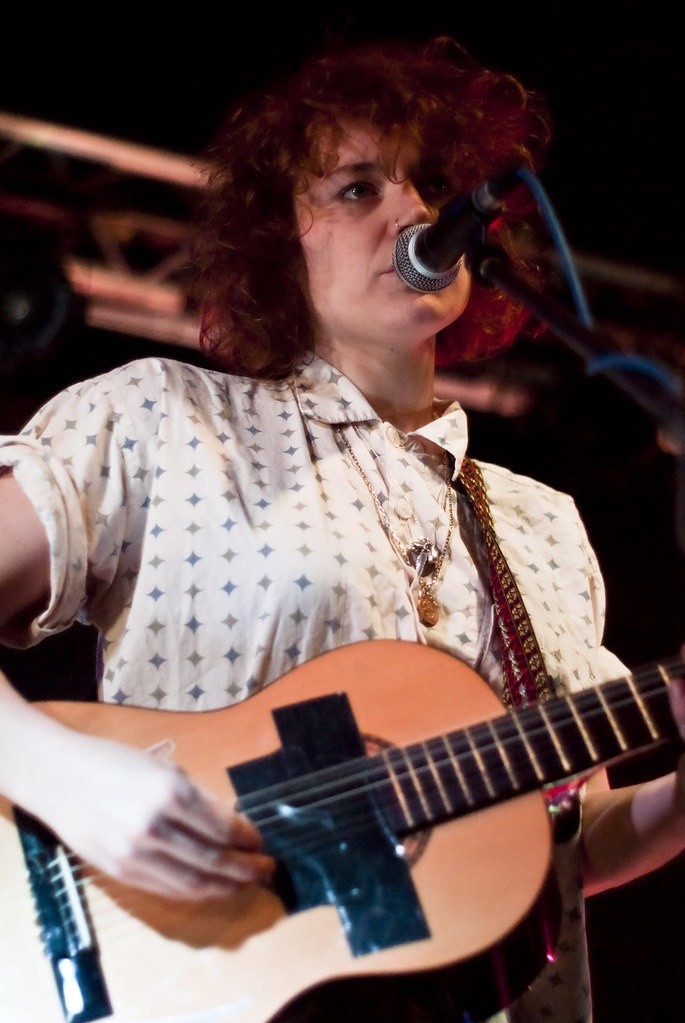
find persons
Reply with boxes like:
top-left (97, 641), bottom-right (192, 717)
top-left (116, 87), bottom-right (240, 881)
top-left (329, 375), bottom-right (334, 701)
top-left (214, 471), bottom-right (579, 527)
top-left (0, 48), bottom-right (685, 1023)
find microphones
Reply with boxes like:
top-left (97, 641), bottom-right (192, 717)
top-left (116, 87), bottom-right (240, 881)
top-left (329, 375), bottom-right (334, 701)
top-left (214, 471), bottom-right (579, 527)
top-left (393, 162), bottom-right (531, 291)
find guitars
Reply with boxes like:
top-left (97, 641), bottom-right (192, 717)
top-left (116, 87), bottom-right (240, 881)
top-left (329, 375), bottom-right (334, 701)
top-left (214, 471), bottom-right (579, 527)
top-left (0, 637), bottom-right (684, 1022)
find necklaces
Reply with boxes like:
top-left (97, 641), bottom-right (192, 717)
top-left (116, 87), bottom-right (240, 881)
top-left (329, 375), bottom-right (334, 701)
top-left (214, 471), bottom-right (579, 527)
top-left (333, 425), bottom-right (455, 627)
